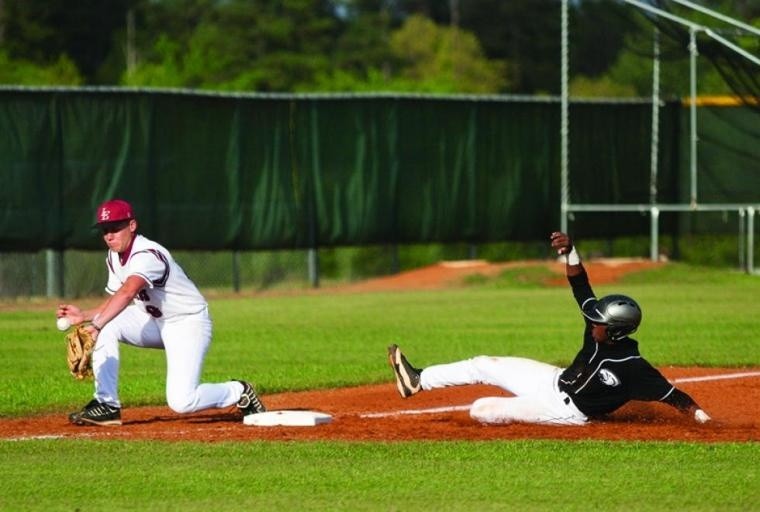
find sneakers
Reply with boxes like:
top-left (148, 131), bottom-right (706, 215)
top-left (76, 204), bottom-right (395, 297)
top-left (232, 378), bottom-right (265, 414)
top-left (70, 400), bottom-right (122, 428)
top-left (388, 343), bottom-right (422, 399)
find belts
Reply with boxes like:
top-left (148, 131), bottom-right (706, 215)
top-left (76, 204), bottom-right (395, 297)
top-left (558, 381), bottom-right (570, 404)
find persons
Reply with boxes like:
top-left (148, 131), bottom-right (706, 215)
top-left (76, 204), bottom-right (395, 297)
top-left (386, 231), bottom-right (723, 430)
top-left (53, 199), bottom-right (265, 428)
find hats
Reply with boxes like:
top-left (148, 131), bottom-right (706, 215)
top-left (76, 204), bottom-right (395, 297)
top-left (90, 199), bottom-right (136, 229)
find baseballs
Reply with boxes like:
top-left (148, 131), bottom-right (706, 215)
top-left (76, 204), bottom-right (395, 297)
top-left (56, 317), bottom-right (71, 331)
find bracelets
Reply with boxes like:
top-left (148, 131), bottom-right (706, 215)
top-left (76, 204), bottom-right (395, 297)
top-left (693, 407), bottom-right (712, 424)
top-left (565, 245), bottom-right (580, 267)
top-left (91, 322), bottom-right (101, 334)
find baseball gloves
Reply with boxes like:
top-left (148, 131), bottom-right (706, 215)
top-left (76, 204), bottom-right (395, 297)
top-left (65, 325), bottom-right (96, 381)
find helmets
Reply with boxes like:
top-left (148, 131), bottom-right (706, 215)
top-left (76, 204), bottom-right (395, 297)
top-left (582, 295), bottom-right (642, 342)
